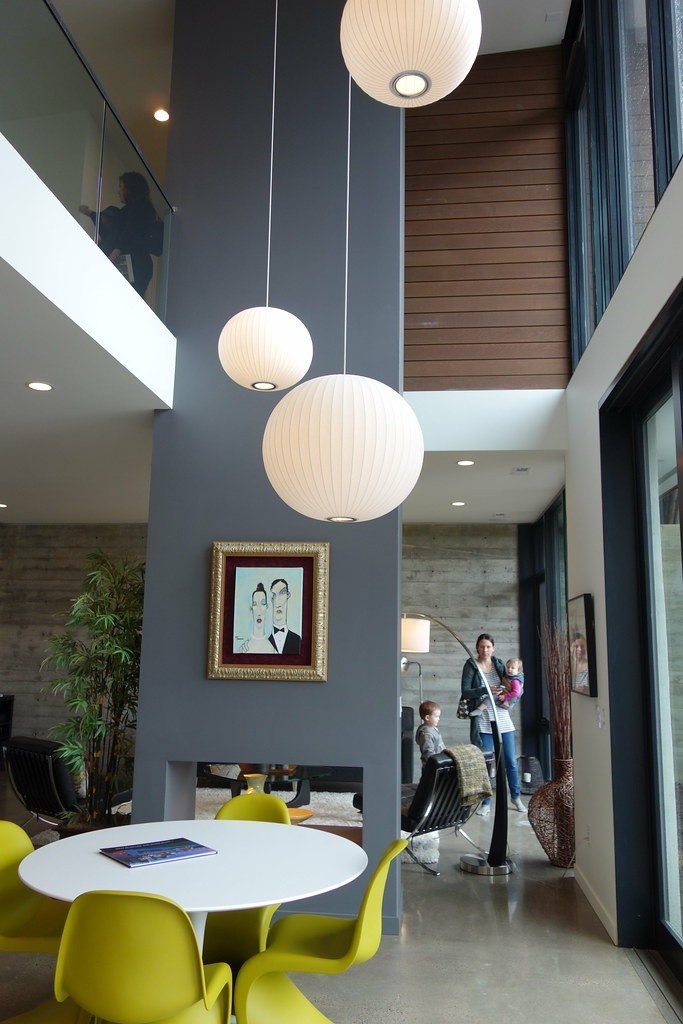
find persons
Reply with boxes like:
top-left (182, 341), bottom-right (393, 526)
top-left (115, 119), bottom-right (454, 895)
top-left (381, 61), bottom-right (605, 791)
top-left (570, 633), bottom-right (590, 693)
top-left (416, 701), bottom-right (447, 774)
top-left (461, 634), bottom-right (528, 813)
top-left (80, 171), bottom-right (158, 300)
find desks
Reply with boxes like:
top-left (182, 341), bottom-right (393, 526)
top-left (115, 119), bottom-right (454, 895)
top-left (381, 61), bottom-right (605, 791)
top-left (18, 819), bottom-right (369, 956)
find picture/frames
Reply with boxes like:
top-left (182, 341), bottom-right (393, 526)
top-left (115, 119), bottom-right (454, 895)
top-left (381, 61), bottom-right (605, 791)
top-left (567, 593), bottom-right (598, 698)
top-left (206, 541), bottom-right (331, 683)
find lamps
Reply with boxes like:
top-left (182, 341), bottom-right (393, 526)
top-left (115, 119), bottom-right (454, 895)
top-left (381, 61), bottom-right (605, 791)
top-left (340, 0), bottom-right (482, 108)
top-left (262, 76), bottom-right (425, 524)
top-left (401, 611), bottom-right (516, 876)
top-left (217, 0), bottom-right (313, 393)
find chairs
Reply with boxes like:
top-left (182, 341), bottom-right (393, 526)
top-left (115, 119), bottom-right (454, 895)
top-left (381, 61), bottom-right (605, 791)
top-left (0, 820), bottom-right (93, 1024)
top-left (401, 744), bottom-right (494, 877)
top-left (233, 837), bottom-right (409, 1024)
top-left (54, 890), bottom-right (233, 1024)
top-left (3, 736), bottom-right (133, 829)
top-left (203, 791), bottom-right (292, 1016)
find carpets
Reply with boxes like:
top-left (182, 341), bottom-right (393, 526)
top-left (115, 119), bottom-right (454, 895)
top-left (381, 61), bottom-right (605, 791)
top-left (106, 787), bottom-right (440, 865)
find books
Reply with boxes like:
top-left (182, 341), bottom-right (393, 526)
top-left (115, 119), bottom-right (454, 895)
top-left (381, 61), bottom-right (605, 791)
top-left (99, 837), bottom-right (218, 868)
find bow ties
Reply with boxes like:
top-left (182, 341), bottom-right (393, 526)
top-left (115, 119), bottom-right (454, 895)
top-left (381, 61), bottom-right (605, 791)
top-left (272, 626), bottom-right (285, 634)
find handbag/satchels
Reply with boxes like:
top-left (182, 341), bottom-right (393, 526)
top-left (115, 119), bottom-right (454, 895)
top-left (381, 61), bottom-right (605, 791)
top-left (456, 694), bottom-right (476, 719)
